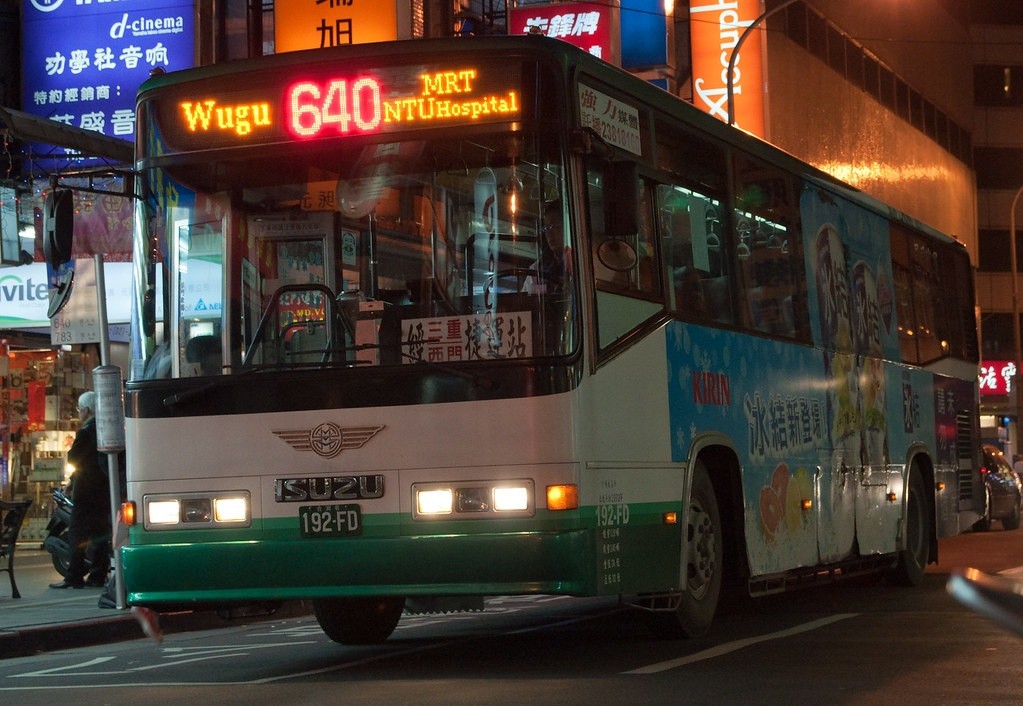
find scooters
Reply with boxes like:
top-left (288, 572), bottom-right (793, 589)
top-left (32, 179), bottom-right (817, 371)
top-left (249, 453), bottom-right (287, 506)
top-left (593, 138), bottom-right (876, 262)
top-left (41, 486), bottom-right (116, 579)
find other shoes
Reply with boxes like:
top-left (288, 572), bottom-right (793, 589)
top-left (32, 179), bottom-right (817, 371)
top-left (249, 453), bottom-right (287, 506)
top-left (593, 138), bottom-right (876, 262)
top-left (49, 579), bottom-right (85, 590)
top-left (945, 566), bottom-right (1023, 634)
top-left (85, 579), bottom-right (104, 586)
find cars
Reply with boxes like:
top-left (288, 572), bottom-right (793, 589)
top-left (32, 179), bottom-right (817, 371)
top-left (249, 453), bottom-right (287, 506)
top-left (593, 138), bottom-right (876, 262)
top-left (973, 441), bottom-right (1023, 531)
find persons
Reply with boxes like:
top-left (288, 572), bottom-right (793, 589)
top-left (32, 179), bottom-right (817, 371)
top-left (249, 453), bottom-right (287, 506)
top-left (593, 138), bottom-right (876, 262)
top-left (49, 390), bottom-right (127, 589)
top-left (646, 221), bottom-right (796, 334)
top-left (522, 193), bottom-right (574, 295)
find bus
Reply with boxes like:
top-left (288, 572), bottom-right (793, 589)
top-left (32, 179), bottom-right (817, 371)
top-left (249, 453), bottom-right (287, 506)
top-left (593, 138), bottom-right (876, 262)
top-left (53, 32), bottom-right (989, 644)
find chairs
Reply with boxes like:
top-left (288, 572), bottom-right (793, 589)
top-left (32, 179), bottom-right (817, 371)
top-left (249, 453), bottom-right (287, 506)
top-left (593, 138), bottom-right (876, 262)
top-left (0, 494), bottom-right (33, 598)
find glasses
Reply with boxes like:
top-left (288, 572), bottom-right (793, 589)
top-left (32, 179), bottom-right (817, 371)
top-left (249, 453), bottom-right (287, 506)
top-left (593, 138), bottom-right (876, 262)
top-left (76, 406), bottom-right (84, 413)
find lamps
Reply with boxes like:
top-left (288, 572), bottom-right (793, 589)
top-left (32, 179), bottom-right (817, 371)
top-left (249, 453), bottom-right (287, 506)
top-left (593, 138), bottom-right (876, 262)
top-left (21, 250), bottom-right (34, 265)
